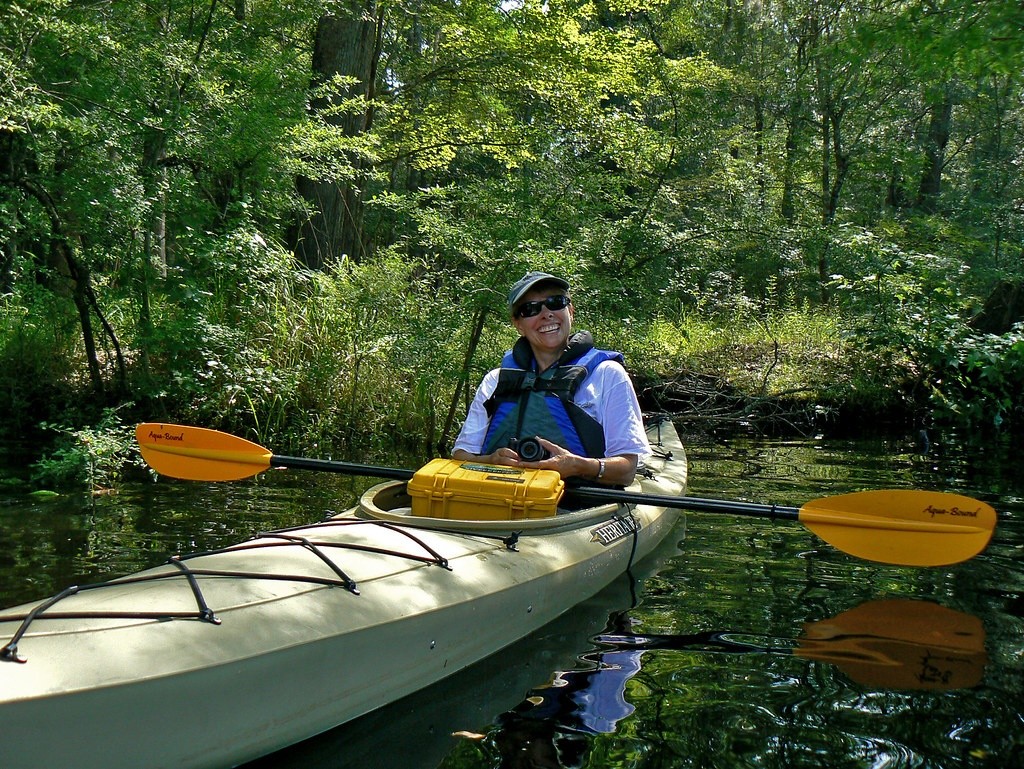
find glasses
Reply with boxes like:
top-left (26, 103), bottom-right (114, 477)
top-left (513, 294), bottom-right (571, 319)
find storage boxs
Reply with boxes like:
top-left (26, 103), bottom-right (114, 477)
top-left (404, 458), bottom-right (565, 520)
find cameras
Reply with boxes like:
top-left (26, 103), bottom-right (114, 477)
top-left (507, 438), bottom-right (551, 463)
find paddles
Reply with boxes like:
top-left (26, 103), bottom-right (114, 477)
top-left (134, 419), bottom-right (997, 567)
top-left (596, 598), bottom-right (987, 689)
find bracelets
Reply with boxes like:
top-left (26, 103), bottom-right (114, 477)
top-left (592, 458), bottom-right (606, 484)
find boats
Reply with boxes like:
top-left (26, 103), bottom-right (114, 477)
top-left (0, 420), bottom-right (689, 769)
top-left (244, 509), bottom-right (689, 769)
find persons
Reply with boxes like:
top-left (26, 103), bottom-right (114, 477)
top-left (450, 270), bottom-right (653, 511)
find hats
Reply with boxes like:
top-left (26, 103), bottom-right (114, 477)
top-left (509, 270), bottom-right (571, 306)
